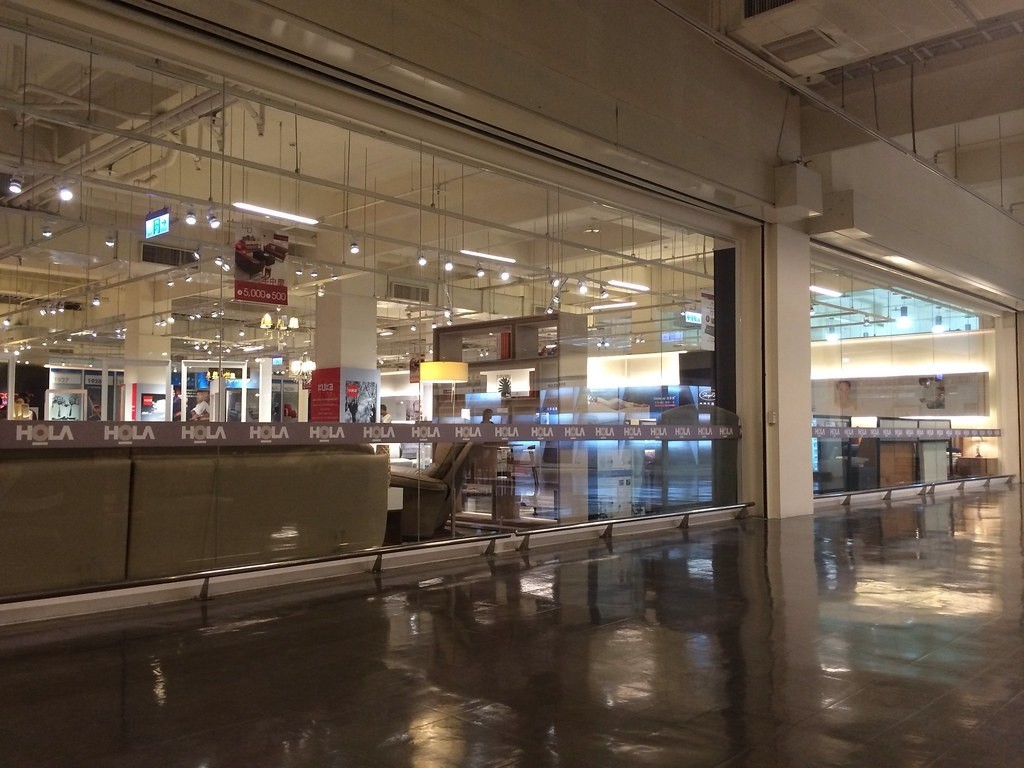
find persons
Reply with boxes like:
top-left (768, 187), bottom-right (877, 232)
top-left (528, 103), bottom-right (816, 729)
top-left (51, 395), bottom-right (80, 421)
top-left (381, 404), bottom-right (391, 423)
top-left (172, 385), bottom-right (211, 422)
top-left (369, 407), bottom-right (376, 423)
top-left (349, 399), bottom-right (358, 422)
top-left (481, 409), bottom-right (494, 424)
top-left (16, 395), bottom-right (37, 420)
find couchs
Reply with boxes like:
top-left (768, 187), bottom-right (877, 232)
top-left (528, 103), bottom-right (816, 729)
top-left (389, 442), bottom-right (472, 538)
top-left (0, 444), bottom-right (389, 605)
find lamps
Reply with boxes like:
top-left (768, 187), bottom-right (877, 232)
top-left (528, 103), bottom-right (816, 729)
top-left (600, 287), bottom-right (608, 298)
top-left (39, 302), bottom-right (64, 315)
top-left (351, 242), bottom-right (359, 254)
top-left (476, 263), bottom-right (485, 278)
top-left (92, 296), bottom-right (100, 306)
top-left (167, 253), bottom-right (228, 286)
top-left (287, 356), bottom-right (316, 384)
top-left (577, 282), bottom-right (588, 293)
top-left (206, 210), bottom-right (221, 228)
top-left (9, 170), bottom-right (23, 193)
top-left (544, 275), bottom-right (560, 315)
top-left (420, 361), bottom-right (469, 384)
top-left (418, 255), bottom-right (427, 265)
top-left (58, 184), bottom-right (73, 201)
top-left (260, 313), bottom-right (299, 335)
top-left (499, 269), bottom-right (509, 280)
top-left (186, 205), bottom-right (196, 224)
top-left (444, 259), bottom-right (453, 271)
